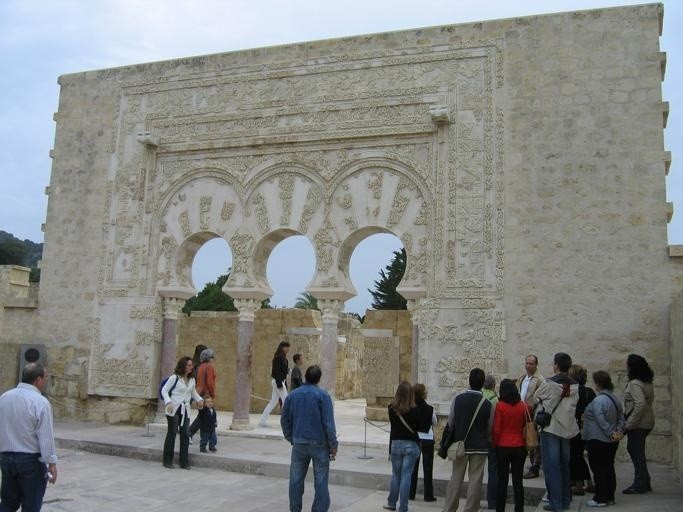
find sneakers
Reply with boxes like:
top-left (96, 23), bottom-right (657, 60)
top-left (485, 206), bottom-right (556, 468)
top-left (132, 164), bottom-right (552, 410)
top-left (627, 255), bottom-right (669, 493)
top-left (200, 444), bottom-right (217, 452)
top-left (623, 484), bottom-right (652, 494)
top-left (383, 504), bottom-right (396, 510)
top-left (587, 500), bottom-right (615, 507)
top-left (571, 486), bottom-right (595, 495)
top-left (523, 471), bottom-right (535, 479)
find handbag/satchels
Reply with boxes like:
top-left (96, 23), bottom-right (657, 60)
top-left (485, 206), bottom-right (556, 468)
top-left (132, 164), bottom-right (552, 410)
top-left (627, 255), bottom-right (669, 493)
top-left (525, 423), bottom-right (539, 450)
top-left (446, 441), bottom-right (465, 462)
top-left (161, 376), bottom-right (178, 398)
top-left (536, 411), bottom-right (551, 426)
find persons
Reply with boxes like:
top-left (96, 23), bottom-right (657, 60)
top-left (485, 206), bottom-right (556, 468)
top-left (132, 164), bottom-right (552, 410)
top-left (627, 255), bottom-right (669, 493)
top-left (197, 395), bottom-right (217, 451)
top-left (189, 349), bottom-right (216, 445)
top-left (291, 353), bottom-right (302, 389)
top-left (622, 355), bottom-right (655, 495)
top-left (190, 346), bottom-right (204, 376)
top-left (383, 381), bottom-right (422, 511)
top-left (0, 362), bottom-right (57, 511)
top-left (408, 383), bottom-right (437, 502)
top-left (515, 355), bottom-right (548, 479)
top-left (159, 354), bottom-right (203, 469)
top-left (437, 368), bottom-right (495, 512)
top-left (480, 375), bottom-right (533, 511)
top-left (566, 364), bottom-right (598, 497)
top-left (533, 352), bottom-right (581, 512)
top-left (280, 365), bottom-right (338, 512)
top-left (259, 341), bottom-right (291, 426)
top-left (584, 371), bottom-right (626, 506)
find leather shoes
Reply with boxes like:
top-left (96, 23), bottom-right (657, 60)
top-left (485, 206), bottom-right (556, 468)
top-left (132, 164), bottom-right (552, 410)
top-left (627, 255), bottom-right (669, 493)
top-left (165, 463), bottom-right (175, 469)
top-left (181, 464), bottom-right (190, 470)
